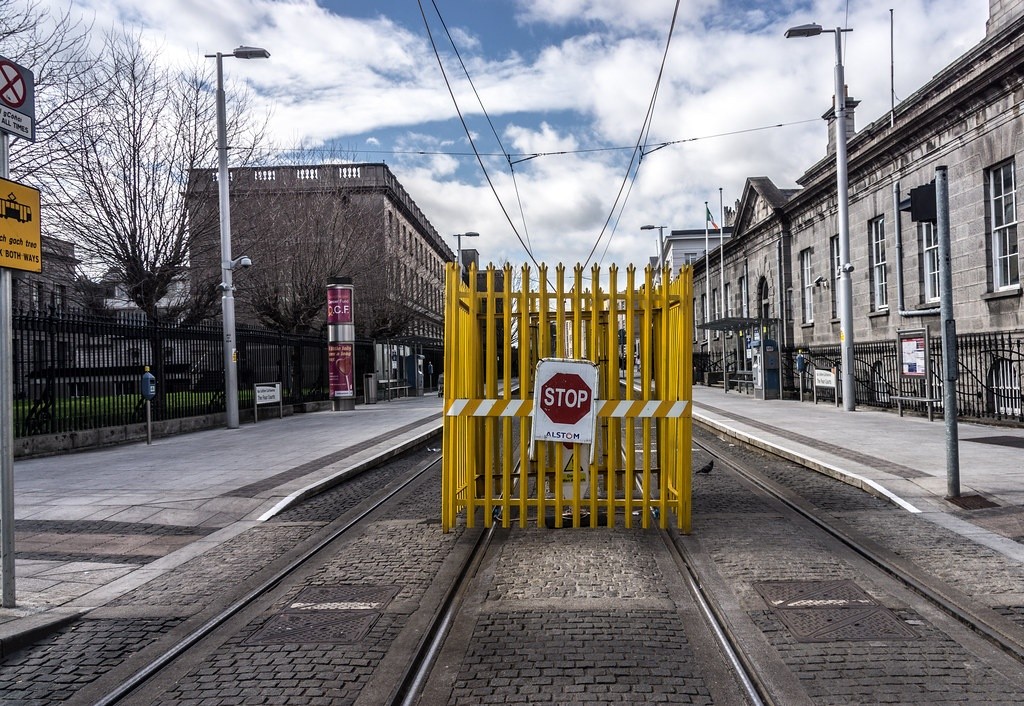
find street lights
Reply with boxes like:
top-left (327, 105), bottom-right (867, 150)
top-left (203, 45), bottom-right (270, 431)
top-left (454, 232), bottom-right (479, 275)
top-left (638, 225), bottom-right (668, 285)
top-left (782, 21), bottom-right (857, 413)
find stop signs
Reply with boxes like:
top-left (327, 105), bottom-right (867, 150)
top-left (529, 355), bottom-right (598, 467)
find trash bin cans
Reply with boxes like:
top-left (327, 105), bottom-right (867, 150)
top-left (363, 372), bottom-right (377, 402)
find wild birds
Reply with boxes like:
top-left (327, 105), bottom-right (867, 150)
top-left (694, 459), bottom-right (714, 476)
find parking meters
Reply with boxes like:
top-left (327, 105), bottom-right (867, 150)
top-left (141, 366), bottom-right (156, 444)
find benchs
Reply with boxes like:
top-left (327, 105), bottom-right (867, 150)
top-left (378, 379), bottom-right (411, 399)
top-left (730, 371), bottom-right (754, 394)
